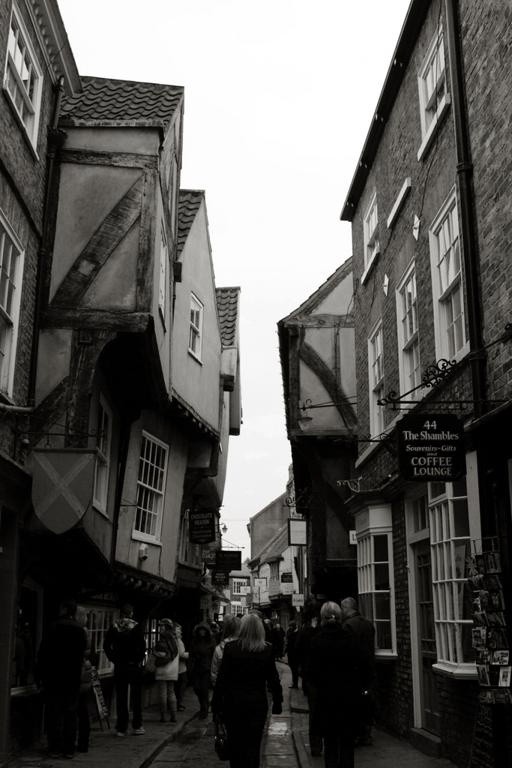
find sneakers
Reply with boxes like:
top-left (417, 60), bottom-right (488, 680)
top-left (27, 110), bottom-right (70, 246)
top-left (114, 727), bottom-right (146, 737)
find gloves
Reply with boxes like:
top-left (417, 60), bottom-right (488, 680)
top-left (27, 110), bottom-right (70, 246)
top-left (271, 702), bottom-right (283, 714)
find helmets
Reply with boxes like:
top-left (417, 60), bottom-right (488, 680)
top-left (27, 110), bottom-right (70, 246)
top-left (156, 618), bottom-right (173, 628)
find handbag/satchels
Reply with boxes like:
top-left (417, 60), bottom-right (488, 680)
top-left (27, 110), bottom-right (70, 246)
top-left (214, 720), bottom-right (231, 763)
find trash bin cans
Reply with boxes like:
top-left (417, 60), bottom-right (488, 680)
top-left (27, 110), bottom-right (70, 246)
top-left (433, 660), bottom-right (478, 765)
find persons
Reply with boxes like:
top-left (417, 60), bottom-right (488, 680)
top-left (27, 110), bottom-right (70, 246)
top-left (212, 616), bottom-right (241, 760)
top-left (37, 602), bottom-right (100, 757)
top-left (145, 618), bottom-right (189, 724)
top-left (187, 621), bottom-right (217, 721)
top-left (211, 614), bottom-right (283, 767)
top-left (305, 601), bottom-right (376, 767)
top-left (264, 598), bottom-right (376, 758)
top-left (103, 606), bottom-right (146, 739)
